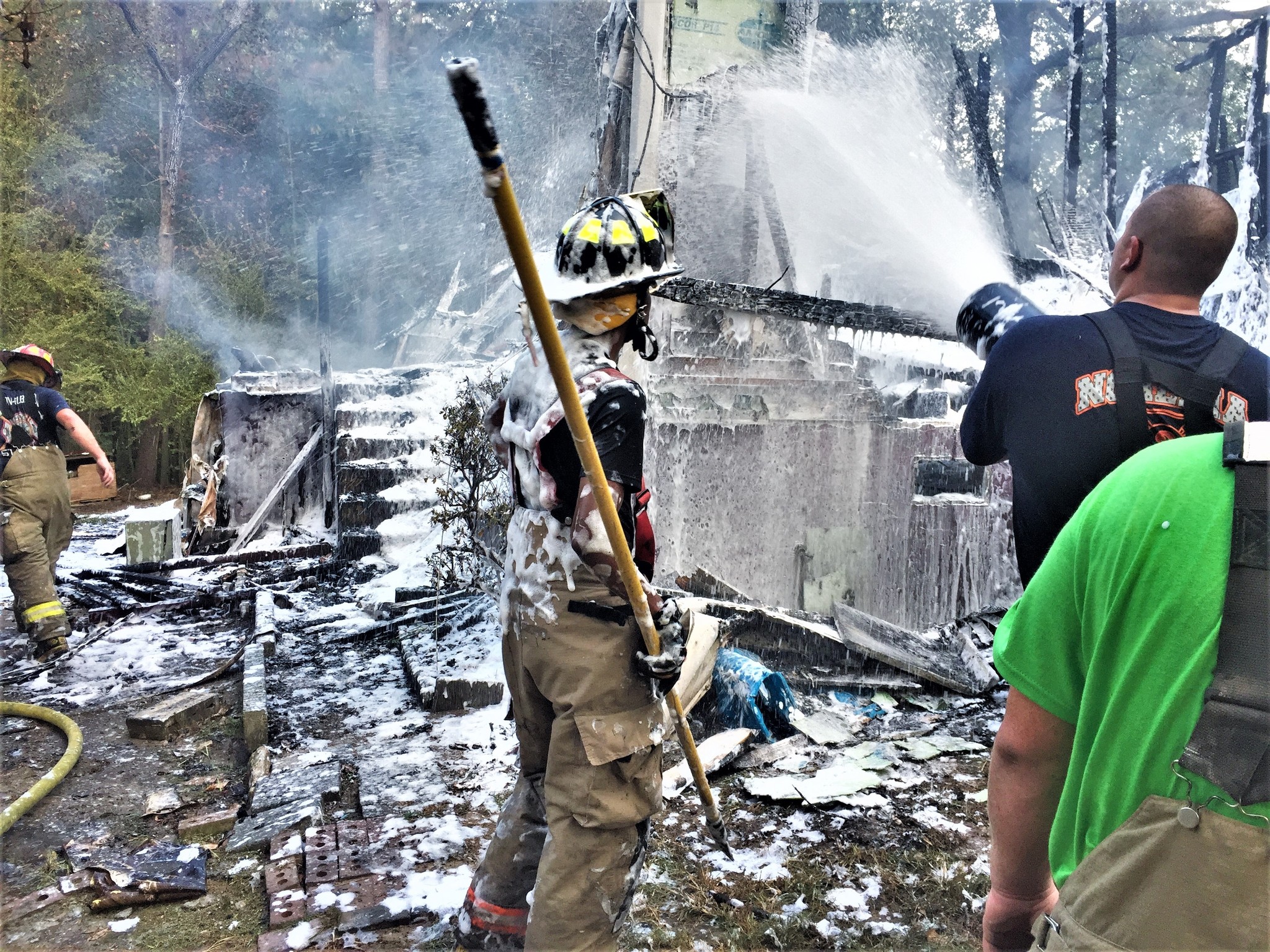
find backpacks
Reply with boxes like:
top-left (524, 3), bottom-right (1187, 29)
top-left (1027, 419), bottom-right (1269, 952)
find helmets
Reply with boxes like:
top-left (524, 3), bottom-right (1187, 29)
top-left (545, 195), bottom-right (686, 305)
top-left (0, 343), bottom-right (64, 390)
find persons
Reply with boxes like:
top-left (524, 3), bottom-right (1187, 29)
top-left (957, 185), bottom-right (1270, 592)
top-left (980, 415), bottom-right (1269, 952)
top-left (418, 189), bottom-right (687, 948)
top-left (0, 341), bottom-right (113, 665)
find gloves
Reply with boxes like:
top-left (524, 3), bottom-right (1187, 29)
top-left (633, 592), bottom-right (688, 704)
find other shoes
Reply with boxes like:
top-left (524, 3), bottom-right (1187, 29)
top-left (32, 635), bottom-right (71, 663)
top-left (451, 919), bottom-right (526, 952)
